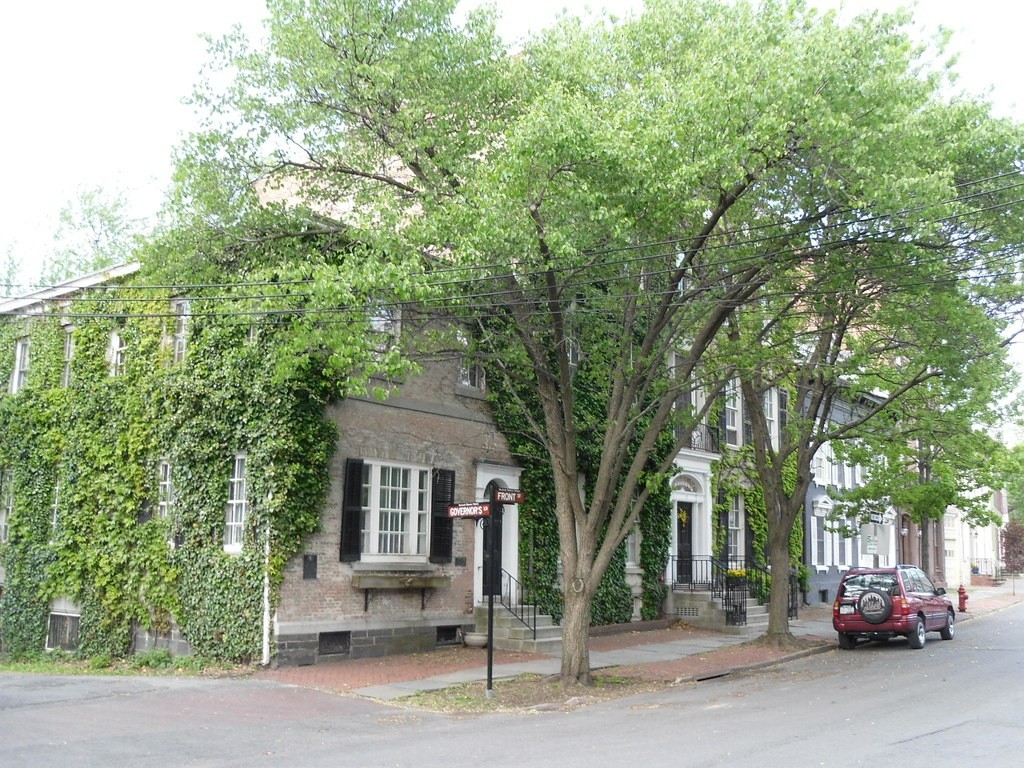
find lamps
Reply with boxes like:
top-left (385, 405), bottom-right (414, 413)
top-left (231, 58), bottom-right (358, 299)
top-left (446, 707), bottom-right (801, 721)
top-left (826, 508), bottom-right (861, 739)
top-left (913, 528), bottom-right (923, 538)
top-left (896, 525), bottom-right (908, 537)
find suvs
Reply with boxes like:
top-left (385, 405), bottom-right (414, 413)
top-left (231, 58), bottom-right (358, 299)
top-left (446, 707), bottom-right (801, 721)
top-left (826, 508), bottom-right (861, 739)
top-left (832, 564), bottom-right (955, 649)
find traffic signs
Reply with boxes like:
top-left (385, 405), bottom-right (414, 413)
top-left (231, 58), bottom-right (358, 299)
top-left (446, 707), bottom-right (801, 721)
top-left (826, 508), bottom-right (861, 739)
top-left (870, 511), bottom-right (883, 524)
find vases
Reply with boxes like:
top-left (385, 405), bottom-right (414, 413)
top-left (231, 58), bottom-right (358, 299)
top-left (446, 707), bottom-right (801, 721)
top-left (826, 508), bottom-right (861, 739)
top-left (465, 633), bottom-right (488, 649)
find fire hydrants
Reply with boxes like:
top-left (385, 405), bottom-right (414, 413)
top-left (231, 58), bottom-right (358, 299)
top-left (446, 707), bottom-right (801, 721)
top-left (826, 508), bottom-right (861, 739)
top-left (957, 584), bottom-right (969, 612)
